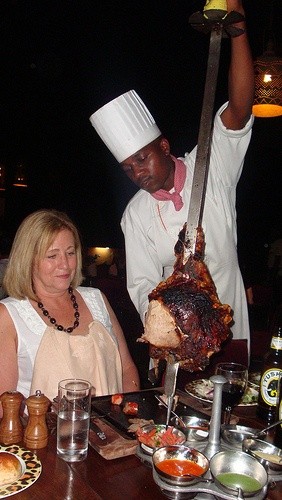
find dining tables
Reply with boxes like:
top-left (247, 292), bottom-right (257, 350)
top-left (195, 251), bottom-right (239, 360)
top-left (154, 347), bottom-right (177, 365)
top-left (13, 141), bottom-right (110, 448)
top-left (0, 386), bottom-right (282, 500)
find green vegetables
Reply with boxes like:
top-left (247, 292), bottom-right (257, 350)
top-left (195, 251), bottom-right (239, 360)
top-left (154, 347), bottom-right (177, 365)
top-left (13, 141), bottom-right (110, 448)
top-left (147, 425), bottom-right (162, 447)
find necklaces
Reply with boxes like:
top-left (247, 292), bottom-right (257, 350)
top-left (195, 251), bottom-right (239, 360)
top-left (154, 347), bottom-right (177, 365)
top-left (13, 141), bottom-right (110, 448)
top-left (36, 286), bottom-right (80, 333)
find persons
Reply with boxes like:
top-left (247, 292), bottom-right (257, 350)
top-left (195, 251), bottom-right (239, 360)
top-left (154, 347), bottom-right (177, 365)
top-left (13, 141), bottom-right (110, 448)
top-left (0, 210), bottom-right (142, 423)
top-left (90, 0), bottom-right (256, 390)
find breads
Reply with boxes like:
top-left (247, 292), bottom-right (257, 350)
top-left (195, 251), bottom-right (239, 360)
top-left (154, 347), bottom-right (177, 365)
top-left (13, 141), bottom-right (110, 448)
top-left (0, 452), bottom-right (22, 486)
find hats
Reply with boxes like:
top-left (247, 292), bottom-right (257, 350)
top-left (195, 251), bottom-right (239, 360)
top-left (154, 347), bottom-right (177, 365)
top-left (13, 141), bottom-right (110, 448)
top-left (89, 88), bottom-right (163, 166)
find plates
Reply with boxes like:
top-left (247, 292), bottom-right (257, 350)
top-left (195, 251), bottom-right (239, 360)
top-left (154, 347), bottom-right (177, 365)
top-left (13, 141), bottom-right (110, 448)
top-left (0, 442), bottom-right (42, 499)
top-left (184, 378), bottom-right (263, 406)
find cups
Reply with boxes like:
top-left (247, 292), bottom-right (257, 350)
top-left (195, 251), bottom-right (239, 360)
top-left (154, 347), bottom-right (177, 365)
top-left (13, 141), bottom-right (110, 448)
top-left (55, 379), bottom-right (94, 461)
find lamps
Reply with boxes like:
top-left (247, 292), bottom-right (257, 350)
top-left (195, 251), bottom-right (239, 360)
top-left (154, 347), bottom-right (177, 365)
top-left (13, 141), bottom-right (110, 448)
top-left (251, 41), bottom-right (282, 118)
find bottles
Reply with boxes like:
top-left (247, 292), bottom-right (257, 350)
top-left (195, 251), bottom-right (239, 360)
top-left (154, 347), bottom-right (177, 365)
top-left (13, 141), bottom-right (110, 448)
top-left (273, 365), bottom-right (282, 448)
top-left (257, 321), bottom-right (282, 422)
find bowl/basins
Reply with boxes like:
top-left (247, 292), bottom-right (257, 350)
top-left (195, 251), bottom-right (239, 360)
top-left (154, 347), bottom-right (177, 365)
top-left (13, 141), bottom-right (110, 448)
top-left (135, 413), bottom-right (282, 500)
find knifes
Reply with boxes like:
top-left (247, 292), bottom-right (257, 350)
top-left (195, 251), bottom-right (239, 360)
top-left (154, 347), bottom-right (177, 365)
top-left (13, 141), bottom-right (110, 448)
top-left (87, 418), bottom-right (109, 445)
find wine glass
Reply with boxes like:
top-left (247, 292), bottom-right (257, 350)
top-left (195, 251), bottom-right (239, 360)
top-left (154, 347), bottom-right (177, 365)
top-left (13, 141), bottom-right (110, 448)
top-left (212, 362), bottom-right (248, 427)
